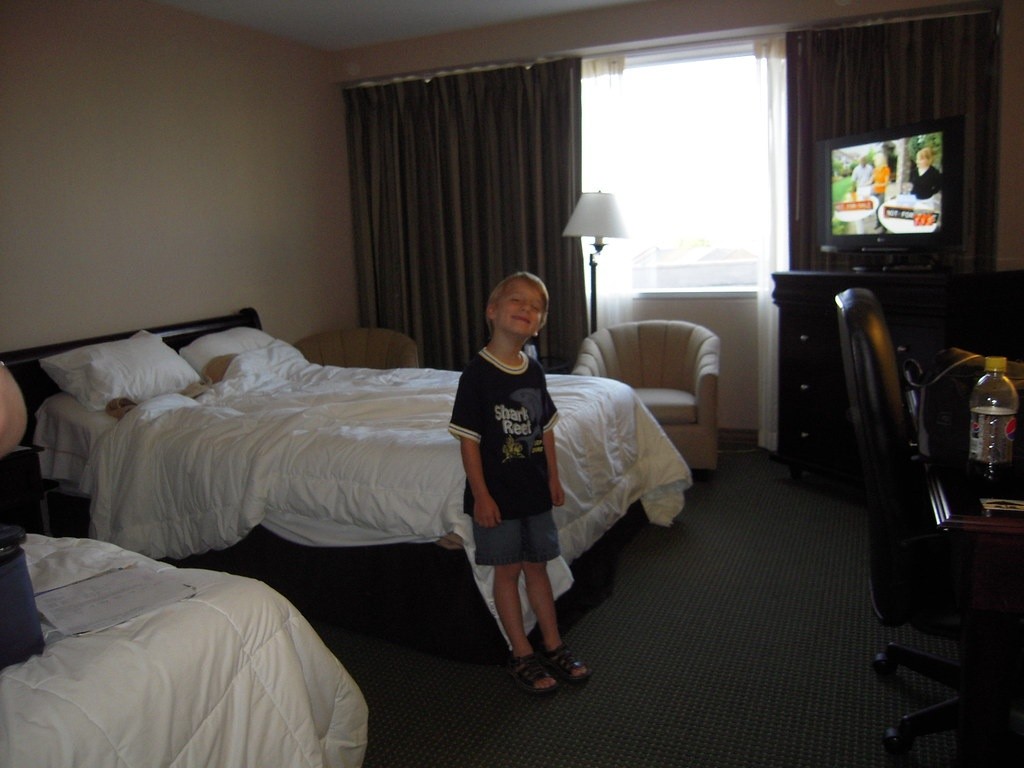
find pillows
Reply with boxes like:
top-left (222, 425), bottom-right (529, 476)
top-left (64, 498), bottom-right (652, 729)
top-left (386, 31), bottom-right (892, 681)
top-left (178, 327), bottom-right (306, 381)
top-left (38, 329), bottom-right (201, 412)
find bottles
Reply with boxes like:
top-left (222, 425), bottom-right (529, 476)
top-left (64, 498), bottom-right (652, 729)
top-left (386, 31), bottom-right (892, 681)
top-left (969, 356), bottom-right (1018, 509)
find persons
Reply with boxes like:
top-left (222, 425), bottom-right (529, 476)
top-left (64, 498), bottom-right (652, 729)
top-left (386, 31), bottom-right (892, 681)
top-left (907, 147), bottom-right (942, 200)
top-left (447, 270), bottom-right (593, 694)
top-left (850, 153), bottom-right (890, 235)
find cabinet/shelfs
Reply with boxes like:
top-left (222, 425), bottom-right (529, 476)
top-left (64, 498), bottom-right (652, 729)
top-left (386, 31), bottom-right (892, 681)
top-left (769, 269), bottom-right (1024, 504)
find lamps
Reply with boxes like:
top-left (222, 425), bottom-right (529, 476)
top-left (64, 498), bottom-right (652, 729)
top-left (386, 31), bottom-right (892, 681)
top-left (561, 191), bottom-right (630, 335)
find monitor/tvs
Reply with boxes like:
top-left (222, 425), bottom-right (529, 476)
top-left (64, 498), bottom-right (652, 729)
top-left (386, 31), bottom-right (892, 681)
top-left (811, 116), bottom-right (964, 272)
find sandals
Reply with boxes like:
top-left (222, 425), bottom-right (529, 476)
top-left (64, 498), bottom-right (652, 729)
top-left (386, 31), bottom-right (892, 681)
top-left (504, 650), bottom-right (559, 693)
top-left (540, 641), bottom-right (593, 680)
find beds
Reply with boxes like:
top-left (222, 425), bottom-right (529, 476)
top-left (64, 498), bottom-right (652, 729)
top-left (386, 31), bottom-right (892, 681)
top-left (0, 307), bottom-right (692, 666)
top-left (0, 525), bottom-right (369, 768)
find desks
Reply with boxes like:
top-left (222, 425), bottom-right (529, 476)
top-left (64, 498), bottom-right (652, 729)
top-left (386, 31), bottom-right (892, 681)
top-left (925, 468), bottom-right (1024, 767)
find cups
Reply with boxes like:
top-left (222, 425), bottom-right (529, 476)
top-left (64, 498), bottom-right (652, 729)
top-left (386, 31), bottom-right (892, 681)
top-left (820, 245), bottom-right (838, 272)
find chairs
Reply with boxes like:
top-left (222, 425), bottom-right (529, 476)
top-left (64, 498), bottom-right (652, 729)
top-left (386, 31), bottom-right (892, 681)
top-left (292, 327), bottom-right (419, 368)
top-left (835, 288), bottom-right (1024, 754)
top-left (571, 320), bottom-right (721, 475)
